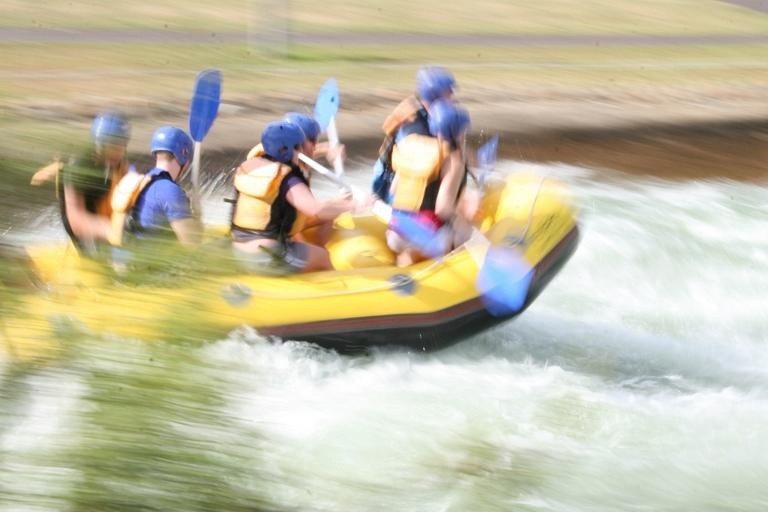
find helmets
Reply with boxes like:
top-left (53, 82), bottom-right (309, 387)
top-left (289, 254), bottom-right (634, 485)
top-left (261, 120), bottom-right (307, 164)
top-left (91, 109), bottom-right (132, 147)
top-left (414, 67), bottom-right (456, 105)
top-left (284, 112), bottom-right (321, 140)
top-left (427, 96), bottom-right (470, 136)
top-left (148, 124), bottom-right (193, 168)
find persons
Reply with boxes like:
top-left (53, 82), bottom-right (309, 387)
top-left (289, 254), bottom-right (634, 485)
top-left (244, 110), bottom-right (354, 246)
top-left (369, 67), bottom-right (459, 222)
top-left (227, 120), bottom-right (379, 277)
top-left (105, 126), bottom-right (205, 281)
top-left (384, 98), bottom-right (484, 267)
top-left (55, 110), bottom-right (129, 266)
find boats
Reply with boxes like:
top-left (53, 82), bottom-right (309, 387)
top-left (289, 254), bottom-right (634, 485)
top-left (29, 161), bottom-right (580, 361)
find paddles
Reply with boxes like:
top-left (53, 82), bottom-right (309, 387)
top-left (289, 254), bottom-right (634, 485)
top-left (298, 152), bottom-right (451, 266)
top-left (463, 226), bottom-right (533, 318)
top-left (477, 133), bottom-right (498, 193)
top-left (314, 77), bottom-right (345, 177)
top-left (188, 69), bottom-right (220, 188)
